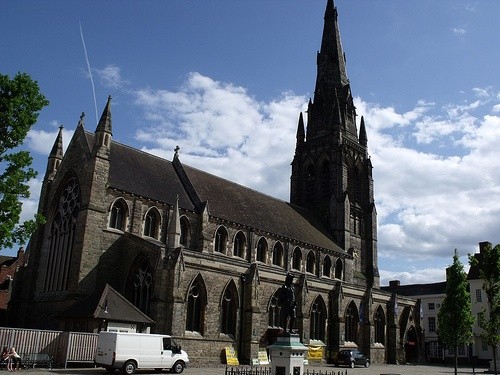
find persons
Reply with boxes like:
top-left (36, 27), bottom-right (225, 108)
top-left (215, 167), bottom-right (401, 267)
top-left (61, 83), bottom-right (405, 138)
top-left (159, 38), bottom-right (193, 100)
top-left (0, 345), bottom-right (14, 372)
top-left (277, 272), bottom-right (298, 335)
top-left (9, 346), bottom-right (22, 371)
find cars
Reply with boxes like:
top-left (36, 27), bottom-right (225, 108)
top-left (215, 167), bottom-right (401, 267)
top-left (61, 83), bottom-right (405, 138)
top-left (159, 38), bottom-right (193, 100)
top-left (334, 350), bottom-right (371, 369)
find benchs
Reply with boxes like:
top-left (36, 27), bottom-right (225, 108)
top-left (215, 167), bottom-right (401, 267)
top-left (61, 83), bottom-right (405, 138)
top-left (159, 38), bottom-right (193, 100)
top-left (0, 352), bottom-right (53, 371)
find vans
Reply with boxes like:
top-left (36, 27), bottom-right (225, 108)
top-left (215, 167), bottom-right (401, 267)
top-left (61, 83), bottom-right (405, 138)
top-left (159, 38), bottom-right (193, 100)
top-left (95, 331), bottom-right (190, 375)
